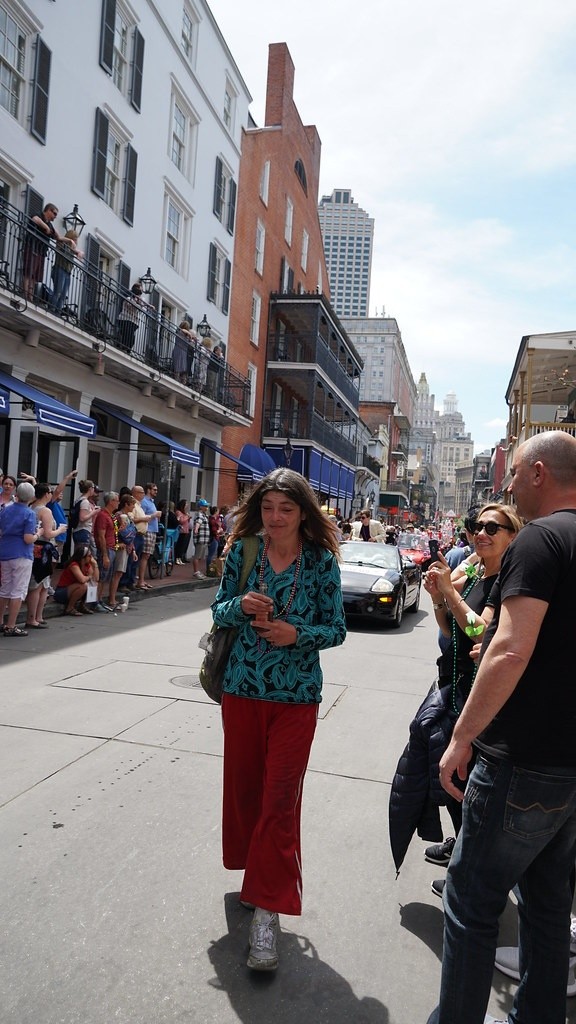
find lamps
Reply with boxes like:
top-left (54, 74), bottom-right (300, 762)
top-left (25, 329), bottom-right (41, 348)
top-left (191, 406), bottom-right (198, 418)
top-left (167, 394), bottom-right (176, 409)
top-left (282, 436), bottom-right (294, 467)
top-left (197, 314), bottom-right (211, 337)
top-left (93, 361), bottom-right (105, 376)
top-left (142, 385), bottom-right (152, 397)
top-left (139, 267), bottom-right (157, 294)
top-left (63, 204), bottom-right (86, 237)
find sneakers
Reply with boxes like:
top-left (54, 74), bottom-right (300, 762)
top-left (494, 945), bottom-right (576, 995)
top-left (246, 912), bottom-right (281, 969)
top-left (424, 837), bottom-right (456, 863)
top-left (432, 879), bottom-right (447, 895)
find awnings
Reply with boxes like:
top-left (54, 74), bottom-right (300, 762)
top-left (238, 442), bottom-right (276, 482)
top-left (88, 401), bottom-right (201, 466)
top-left (202, 439), bottom-right (264, 481)
top-left (0, 372), bottom-right (97, 438)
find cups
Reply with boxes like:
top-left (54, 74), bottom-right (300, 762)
top-left (123, 597), bottom-right (130, 609)
top-left (95, 506), bottom-right (100, 510)
top-left (121, 604), bottom-right (127, 612)
top-left (60, 524), bottom-right (67, 533)
top-left (120, 514), bottom-right (127, 524)
top-left (156, 511), bottom-right (161, 517)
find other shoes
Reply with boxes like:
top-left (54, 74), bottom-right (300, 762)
top-left (175, 558), bottom-right (185, 565)
top-left (120, 581), bottom-right (153, 593)
top-left (24, 623), bottom-right (49, 629)
top-left (3, 625), bottom-right (28, 637)
top-left (193, 570), bottom-right (205, 579)
top-left (69, 603), bottom-right (122, 615)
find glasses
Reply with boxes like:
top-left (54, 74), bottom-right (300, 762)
top-left (47, 490), bottom-right (54, 495)
top-left (358, 515), bottom-right (368, 520)
top-left (467, 522), bottom-right (515, 536)
top-left (51, 210), bottom-right (58, 217)
top-left (152, 489), bottom-right (157, 491)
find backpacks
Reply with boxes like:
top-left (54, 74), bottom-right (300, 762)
top-left (117, 523), bottom-right (136, 544)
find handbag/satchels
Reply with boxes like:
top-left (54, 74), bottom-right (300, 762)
top-left (199, 536), bottom-right (257, 703)
top-left (86, 579), bottom-right (97, 603)
top-left (71, 498), bottom-right (87, 528)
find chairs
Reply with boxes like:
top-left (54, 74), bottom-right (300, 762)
top-left (1, 260), bottom-right (233, 409)
top-left (265, 418), bottom-right (282, 437)
top-left (274, 347), bottom-right (291, 361)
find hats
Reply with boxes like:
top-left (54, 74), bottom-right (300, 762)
top-left (197, 499), bottom-right (210, 507)
top-left (94, 485), bottom-right (104, 493)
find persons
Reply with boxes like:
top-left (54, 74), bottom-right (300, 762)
top-left (423, 504), bottom-right (524, 897)
top-left (496, 893), bottom-right (576, 996)
top-left (210, 468), bottom-right (348, 970)
top-left (0, 468), bottom-right (484, 635)
top-left (51, 230), bottom-right (84, 315)
top-left (428, 430), bottom-right (576, 1023)
top-left (22, 203), bottom-right (76, 301)
top-left (117, 283), bottom-right (155, 354)
top-left (172, 321), bottom-right (224, 400)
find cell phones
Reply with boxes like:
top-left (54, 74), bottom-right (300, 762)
top-left (428, 539), bottom-right (439, 563)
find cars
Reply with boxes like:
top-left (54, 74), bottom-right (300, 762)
top-left (332, 534), bottom-right (431, 628)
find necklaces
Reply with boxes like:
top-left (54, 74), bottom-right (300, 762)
top-left (256, 537), bottom-right (306, 654)
top-left (453, 568), bottom-right (491, 717)
top-left (105, 507), bottom-right (119, 550)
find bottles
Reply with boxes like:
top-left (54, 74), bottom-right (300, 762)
top-left (251, 583), bottom-right (273, 633)
